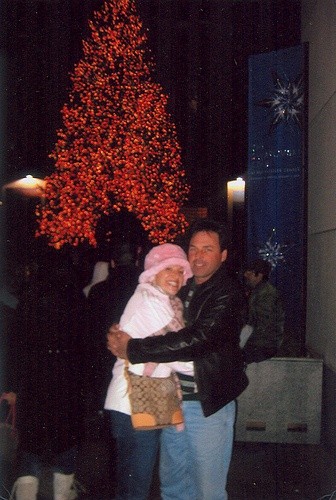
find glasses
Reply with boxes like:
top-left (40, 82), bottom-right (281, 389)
top-left (242, 269), bottom-right (258, 275)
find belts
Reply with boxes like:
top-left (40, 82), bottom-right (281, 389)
top-left (182, 393), bottom-right (201, 401)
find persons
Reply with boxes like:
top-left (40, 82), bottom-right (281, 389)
top-left (74, 249), bottom-right (149, 298)
top-left (88, 235), bottom-right (143, 500)
top-left (106, 223), bottom-right (249, 500)
top-left (242, 260), bottom-right (281, 363)
top-left (6, 246), bottom-right (95, 500)
top-left (104, 244), bottom-right (194, 500)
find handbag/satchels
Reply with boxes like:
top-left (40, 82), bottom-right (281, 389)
top-left (124, 367), bottom-right (185, 431)
top-left (0, 397), bottom-right (19, 461)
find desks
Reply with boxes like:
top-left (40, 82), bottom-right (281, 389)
top-left (233, 344), bottom-right (325, 445)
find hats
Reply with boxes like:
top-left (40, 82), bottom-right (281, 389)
top-left (138, 244), bottom-right (193, 287)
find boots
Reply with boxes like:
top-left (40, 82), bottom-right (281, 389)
top-left (53, 472), bottom-right (78, 500)
top-left (9, 475), bottom-right (39, 500)
